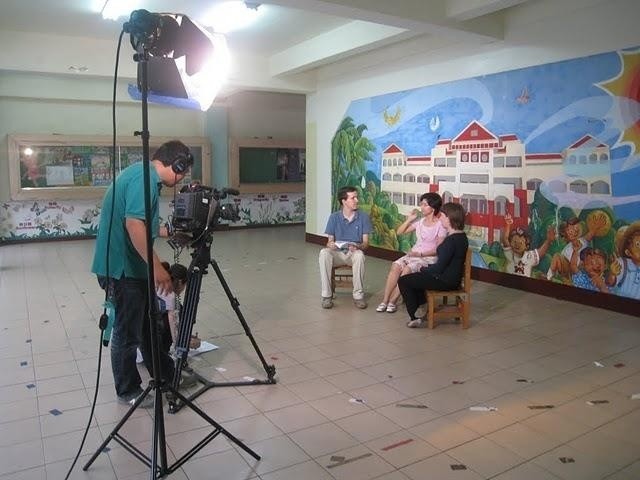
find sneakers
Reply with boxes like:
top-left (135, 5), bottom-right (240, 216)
top-left (414, 303), bottom-right (428, 318)
top-left (387, 304), bottom-right (397, 312)
top-left (177, 375), bottom-right (197, 390)
top-left (406, 319), bottom-right (424, 329)
top-left (376, 303), bottom-right (387, 312)
top-left (322, 297), bottom-right (333, 308)
top-left (117, 391), bottom-right (155, 408)
top-left (353, 299), bottom-right (367, 308)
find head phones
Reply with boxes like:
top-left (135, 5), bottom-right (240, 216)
top-left (172, 158), bottom-right (188, 173)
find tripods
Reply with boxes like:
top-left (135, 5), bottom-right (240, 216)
top-left (167, 261), bottom-right (276, 414)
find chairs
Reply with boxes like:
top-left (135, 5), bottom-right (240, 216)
top-left (332, 266), bottom-right (354, 296)
top-left (426, 248), bottom-right (472, 329)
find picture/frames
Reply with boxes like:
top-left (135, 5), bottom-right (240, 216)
top-left (7, 134), bottom-right (210, 202)
top-left (229, 138), bottom-right (306, 193)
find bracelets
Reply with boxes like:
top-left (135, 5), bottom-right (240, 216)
top-left (420, 252), bottom-right (422, 257)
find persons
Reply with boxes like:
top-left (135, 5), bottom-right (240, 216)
top-left (319, 186), bottom-right (373, 308)
top-left (376, 193), bottom-right (447, 313)
top-left (397, 202), bottom-right (467, 328)
top-left (501, 211), bottom-right (640, 301)
top-left (94, 140), bottom-right (196, 412)
top-left (134, 262), bottom-right (201, 363)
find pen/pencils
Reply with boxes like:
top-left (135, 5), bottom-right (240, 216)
top-left (194, 332), bottom-right (198, 350)
